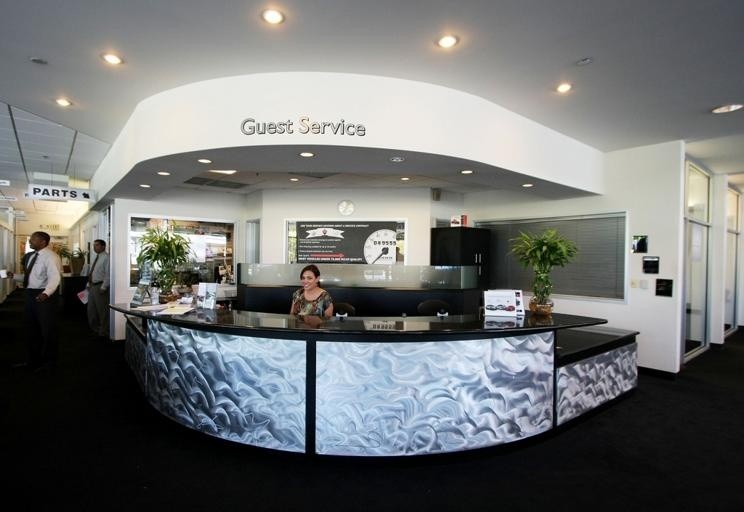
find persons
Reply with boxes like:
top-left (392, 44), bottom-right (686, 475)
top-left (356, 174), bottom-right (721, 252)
top-left (80, 239), bottom-right (110, 346)
top-left (294, 316), bottom-right (332, 329)
top-left (289, 263), bottom-right (336, 316)
top-left (6, 231), bottom-right (65, 375)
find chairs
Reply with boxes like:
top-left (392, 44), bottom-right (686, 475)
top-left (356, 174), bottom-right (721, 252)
top-left (418, 299), bottom-right (450, 316)
top-left (333, 302), bottom-right (356, 318)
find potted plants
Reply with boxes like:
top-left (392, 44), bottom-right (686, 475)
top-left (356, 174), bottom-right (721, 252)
top-left (136, 219), bottom-right (196, 303)
top-left (506, 225), bottom-right (579, 316)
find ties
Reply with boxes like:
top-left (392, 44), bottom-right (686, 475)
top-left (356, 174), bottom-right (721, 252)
top-left (23, 253), bottom-right (38, 289)
top-left (88, 253), bottom-right (98, 286)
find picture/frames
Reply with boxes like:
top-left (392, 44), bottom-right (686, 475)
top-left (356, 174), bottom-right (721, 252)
top-left (631, 234), bottom-right (673, 297)
top-left (283, 217), bottom-right (409, 266)
